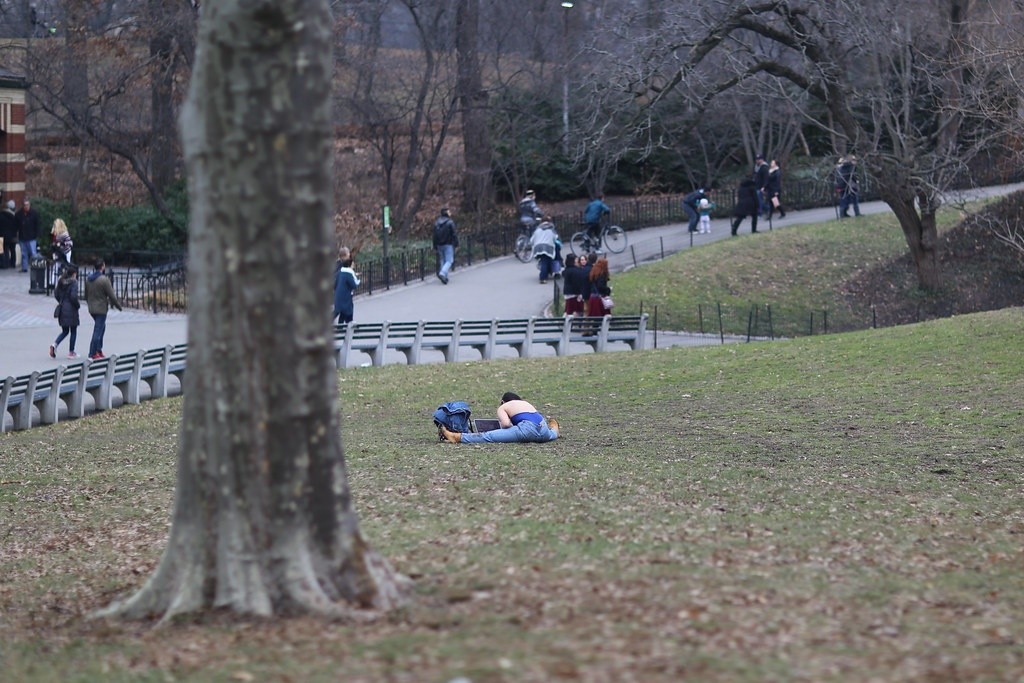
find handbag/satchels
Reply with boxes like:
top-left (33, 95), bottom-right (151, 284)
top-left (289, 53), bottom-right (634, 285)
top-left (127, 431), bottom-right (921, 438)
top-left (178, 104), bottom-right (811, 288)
top-left (53, 304), bottom-right (63, 317)
top-left (602, 295), bottom-right (614, 311)
top-left (772, 196), bottom-right (780, 208)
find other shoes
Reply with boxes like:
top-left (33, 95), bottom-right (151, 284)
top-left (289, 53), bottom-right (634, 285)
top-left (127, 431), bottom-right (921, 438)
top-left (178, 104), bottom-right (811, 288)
top-left (731, 229), bottom-right (737, 236)
top-left (89, 354), bottom-right (104, 362)
top-left (700, 230), bottom-right (705, 234)
top-left (441, 426), bottom-right (456, 444)
top-left (779, 212), bottom-right (787, 219)
top-left (49, 344), bottom-right (58, 358)
top-left (707, 230), bottom-right (711, 233)
top-left (540, 280), bottom-right (548, 285)
top-left (438, 273), bottom-right (449, 285)
top-left (98, 352), bottom-right (107, 358)
top-left (594, 250), bottom-right (603, 255)
top-left (66, 352), bottom-right (81, 359)
top-left (687, 231), bottom-right (699, 235)
top-left (548, 417), bottom-right (559, 431)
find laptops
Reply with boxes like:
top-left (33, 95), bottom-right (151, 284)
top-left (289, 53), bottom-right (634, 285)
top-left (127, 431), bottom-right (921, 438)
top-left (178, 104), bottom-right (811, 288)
top-left (474, 419), bottom-right (501, 433)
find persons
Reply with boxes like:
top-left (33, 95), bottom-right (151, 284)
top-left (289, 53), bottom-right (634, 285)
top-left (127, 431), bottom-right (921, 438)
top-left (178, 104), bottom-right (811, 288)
top-left (334, 260), bottom-right (360, 330)
top-left (731, 153), bottom-right (786, 235)
top-left (0, 200), bottom-right (17, 268)
top-left (441, 392), bottom-right (560, 443)
top-left (582, 250), bottom-right (598, 337)
top-left (432, 208), bottom-right (460, 285)
top-left (587, 256), bottom-right (613, 334)
top-left (50, 267), bottom-right (80, 359)
top-left (583, 192), bottom-right (610, 254)
top-left (332, 246), bottom-right (362, 322)
top-left (580, 255), bottom-right (587, 267)
top-left (48, 218), bottom-right (72, 288)
top-left (84, 259), bottom-right (123, 360)
top-left (832, 153), bottom-right (861, 219)
top-left (15, 200), bottom-right (40, 272)
top-left (518, 190), bottom-right (550, 235)
top-left (682, 186), bottom-right (715, 235)
top-left (529, 216), bottom-right (562, 284)
top-left (562, 253), bottom-right (586, 328)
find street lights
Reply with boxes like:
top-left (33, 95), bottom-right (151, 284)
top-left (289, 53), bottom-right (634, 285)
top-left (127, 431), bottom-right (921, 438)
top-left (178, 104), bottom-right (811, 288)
top-left (561, 2), bottom-right (573, 158)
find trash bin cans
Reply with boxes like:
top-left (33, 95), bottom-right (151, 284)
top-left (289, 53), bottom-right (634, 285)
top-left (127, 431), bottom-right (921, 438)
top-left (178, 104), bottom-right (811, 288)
top-left (28, 256), bottom-right (47, 294)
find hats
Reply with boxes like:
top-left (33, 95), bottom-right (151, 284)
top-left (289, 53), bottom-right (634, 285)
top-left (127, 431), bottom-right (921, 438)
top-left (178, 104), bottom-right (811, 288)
top-left (755, 153), bottom-right (764, 160)
top-left (526, 190), bottom-right (536, 197)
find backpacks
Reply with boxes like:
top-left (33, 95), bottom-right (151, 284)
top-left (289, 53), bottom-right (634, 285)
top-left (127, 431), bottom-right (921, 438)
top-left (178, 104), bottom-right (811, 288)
top-left (436, 218), bottom-right (451, 246)
top-left (432, 400), bottom-right (475, 434)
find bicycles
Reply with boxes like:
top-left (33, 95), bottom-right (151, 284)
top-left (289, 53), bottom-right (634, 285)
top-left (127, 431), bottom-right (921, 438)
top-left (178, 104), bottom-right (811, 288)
top-left (570, 214), bottom-right (627, 257)
top-left (514, 210), bottom-right (536, 263)
